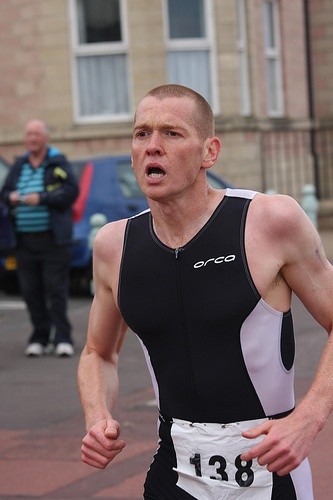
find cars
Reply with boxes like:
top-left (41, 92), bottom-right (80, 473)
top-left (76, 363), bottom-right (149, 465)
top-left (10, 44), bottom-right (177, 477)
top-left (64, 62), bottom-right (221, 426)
top-left (0, 153), bottom-right (234, 298)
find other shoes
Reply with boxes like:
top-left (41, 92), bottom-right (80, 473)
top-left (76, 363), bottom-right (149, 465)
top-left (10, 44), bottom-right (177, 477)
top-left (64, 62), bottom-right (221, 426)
top-left (55, 343), bottom-right (74, 356)
top-left (26, 342), bottom-right (53, 355)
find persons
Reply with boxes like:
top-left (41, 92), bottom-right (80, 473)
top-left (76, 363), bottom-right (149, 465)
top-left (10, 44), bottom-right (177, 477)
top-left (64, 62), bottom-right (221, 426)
top-left (73, 82), bottom-right (333, 500)
top-left (0, 117), bottom-right (82, 356)
